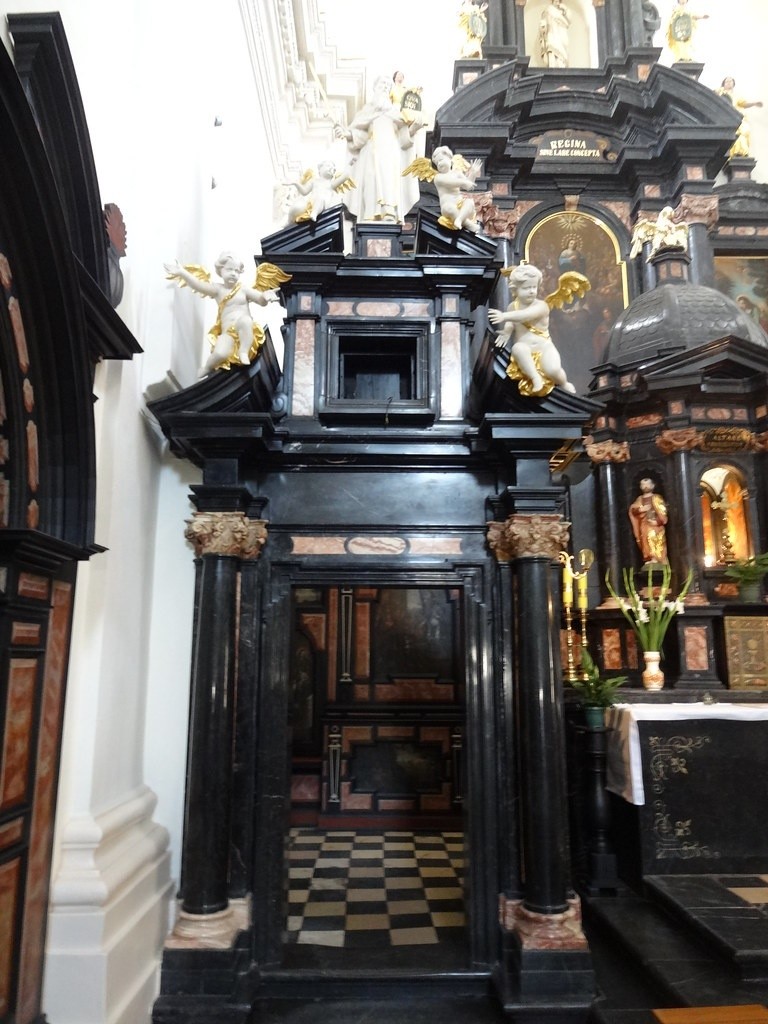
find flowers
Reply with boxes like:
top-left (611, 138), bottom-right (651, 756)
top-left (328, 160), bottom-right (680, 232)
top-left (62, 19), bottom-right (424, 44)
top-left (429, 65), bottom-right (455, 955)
top-left (604, 556), bottom-right (694, 652)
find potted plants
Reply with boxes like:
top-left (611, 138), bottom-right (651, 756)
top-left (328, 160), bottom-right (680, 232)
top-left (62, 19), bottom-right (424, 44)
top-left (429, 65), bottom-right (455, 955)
top-left (562, 642), bottom-right (631, 729)
top-left (724, 552), bottom-right (768, 603)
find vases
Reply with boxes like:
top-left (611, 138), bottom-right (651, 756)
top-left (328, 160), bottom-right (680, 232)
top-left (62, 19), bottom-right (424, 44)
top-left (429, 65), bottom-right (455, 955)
top-left (641, 652), bottom-right (665, 691)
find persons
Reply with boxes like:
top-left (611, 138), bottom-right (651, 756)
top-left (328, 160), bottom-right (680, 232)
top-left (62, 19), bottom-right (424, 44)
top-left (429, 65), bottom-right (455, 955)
top-left (161, 251), bottom-right (281, 378)
top-left (487, 264), bottom-right (577, 395)
top-left (536, 0), bottom-right (573, 69)
top-left (453, 0), bottom-right (489, 61)
top-left (278, 158), bottom-right (353, 229)
top-left (389, 70), bottom-right (423, 106)
top-left (431, 146), bottom-right (483, 234)
top-left (331, 69), bottom-right (425, 221)
top-left (714, 74), bottom-right (763, 157)
top-left (665, 0), bottom-right (709, 63)
top-left (628, 477), bottom-right (670, 566)
